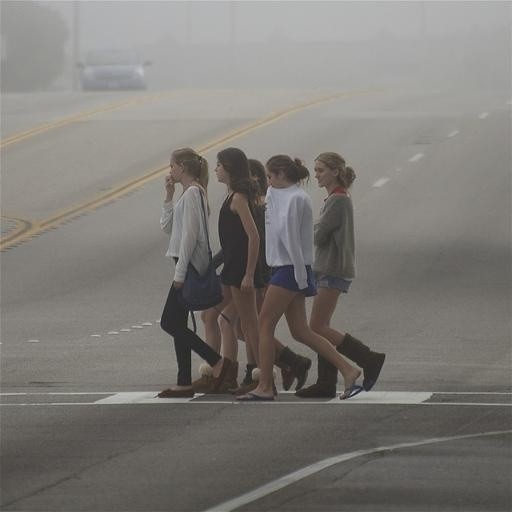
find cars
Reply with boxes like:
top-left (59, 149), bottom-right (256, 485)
top-left (77, 47), bottom-right (152, 91)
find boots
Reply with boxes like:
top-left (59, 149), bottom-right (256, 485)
top-left (157, 333), bottom-right (385, 400)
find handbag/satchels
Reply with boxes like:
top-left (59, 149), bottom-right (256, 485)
top-left (177, 251), bottom-right (225, 311)
